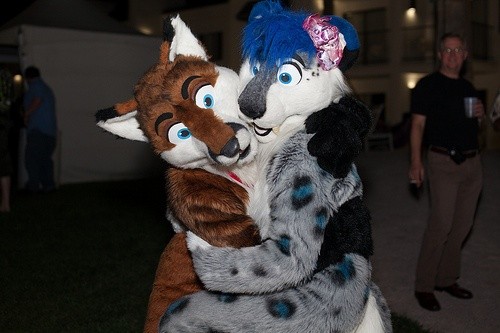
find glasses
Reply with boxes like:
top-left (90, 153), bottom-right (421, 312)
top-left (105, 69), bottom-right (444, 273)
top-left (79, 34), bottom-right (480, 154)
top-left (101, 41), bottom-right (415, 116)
top-left (442, 46), bottom-right (463, 53)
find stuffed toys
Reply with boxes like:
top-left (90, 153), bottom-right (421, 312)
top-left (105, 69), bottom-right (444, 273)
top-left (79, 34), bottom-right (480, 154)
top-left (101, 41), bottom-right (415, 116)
top-left (96, 13), bottom-right (374, 332)
top-left (159, 0), bottom-right (392, 332)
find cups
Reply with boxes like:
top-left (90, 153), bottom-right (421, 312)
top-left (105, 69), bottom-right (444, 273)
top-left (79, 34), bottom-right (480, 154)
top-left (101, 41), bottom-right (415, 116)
top-left (464, 97), bottom-right (478, 118)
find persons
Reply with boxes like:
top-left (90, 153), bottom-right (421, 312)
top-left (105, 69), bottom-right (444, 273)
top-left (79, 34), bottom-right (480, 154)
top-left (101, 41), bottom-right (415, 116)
top-left (22, 66), bottom-right (57, 201)
top-left (409, 32), bottom-right (488, 312)
top-left (0, 70), bottom-right (16, 212)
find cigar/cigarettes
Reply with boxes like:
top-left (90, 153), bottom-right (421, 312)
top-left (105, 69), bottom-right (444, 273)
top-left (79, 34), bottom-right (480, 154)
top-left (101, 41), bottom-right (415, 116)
top-left (410, 180), bottom-right (421, 183)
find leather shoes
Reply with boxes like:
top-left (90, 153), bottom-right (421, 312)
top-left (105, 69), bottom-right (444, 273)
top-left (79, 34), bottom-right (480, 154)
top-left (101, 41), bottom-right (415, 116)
top-left (436, 282), bottom-right (474, 299)
top-left (414, 291), bottom-right (441, 311)
